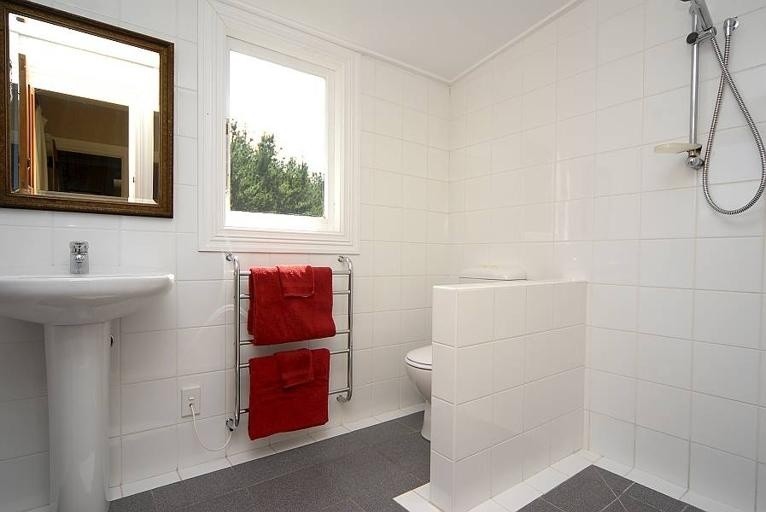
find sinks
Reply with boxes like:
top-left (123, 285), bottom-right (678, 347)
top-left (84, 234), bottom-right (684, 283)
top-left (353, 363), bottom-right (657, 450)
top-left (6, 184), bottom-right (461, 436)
top-left (1, 274), bottom-right (175, 327)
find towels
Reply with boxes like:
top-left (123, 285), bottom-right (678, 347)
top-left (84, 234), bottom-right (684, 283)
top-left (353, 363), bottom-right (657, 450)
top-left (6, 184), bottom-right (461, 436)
top-left (249, 347), bottom-right (330, 441)
top-left (275, 349), bottom-right (315, 388)
top-left (277, 265), bottom-right (315, 299)
top-left (246, 266), bottom-right (337, 346)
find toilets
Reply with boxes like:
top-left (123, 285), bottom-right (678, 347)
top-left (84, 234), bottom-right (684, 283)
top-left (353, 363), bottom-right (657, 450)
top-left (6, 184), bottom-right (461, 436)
top-left (405, 269), bottom-right (528, 442)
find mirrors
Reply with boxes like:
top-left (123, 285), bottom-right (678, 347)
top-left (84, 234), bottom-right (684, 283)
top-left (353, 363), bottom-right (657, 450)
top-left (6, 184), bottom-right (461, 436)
top-left (0, 0), bottom-right (175, 220)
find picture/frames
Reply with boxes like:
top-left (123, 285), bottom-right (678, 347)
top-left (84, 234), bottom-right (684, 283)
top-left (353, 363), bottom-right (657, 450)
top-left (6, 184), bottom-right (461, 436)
top-left (195, 0), bottom-right (364, 256)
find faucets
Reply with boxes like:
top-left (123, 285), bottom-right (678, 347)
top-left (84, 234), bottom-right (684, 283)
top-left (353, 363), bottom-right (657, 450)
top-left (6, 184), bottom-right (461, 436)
top-left (71, 242), bottom-right (91, 274)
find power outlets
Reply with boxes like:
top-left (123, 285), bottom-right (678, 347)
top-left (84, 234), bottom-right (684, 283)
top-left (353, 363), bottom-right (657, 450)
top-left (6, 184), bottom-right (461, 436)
top-left (181, 387), bottom-right (202, 418)
